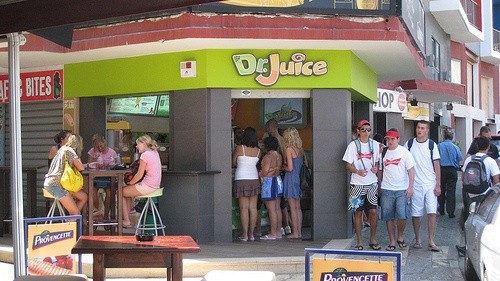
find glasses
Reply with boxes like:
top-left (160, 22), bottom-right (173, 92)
top-left (360, 128), bottom-right (371, 132)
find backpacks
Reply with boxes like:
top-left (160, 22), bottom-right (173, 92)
top-left (462, 154), bottom-right (491, 194)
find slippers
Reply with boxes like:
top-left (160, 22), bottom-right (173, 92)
top-left (427, 243), bottom-right (440, 251)
top-left (386, 244), bottom-right (395, 251)
top-left (414, 242), bottom-right (422, 248)
top-left (368, 242), bottom-right (381, 250)
top-left (259, 235), bottom-right (282, 240)
top-left (355, 245), bottom-right (363, 251)
top-left (396, 240), bottom-right (406, 248)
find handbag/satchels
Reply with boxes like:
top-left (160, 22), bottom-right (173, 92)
top-left (124, 160), bottom-right (145, 185)
top-left (61, 147), bottom-right (84, 193)
top-left (300, 163), bottom-right (310, 191)
top-left (111, 164), bottom-right (125, 170)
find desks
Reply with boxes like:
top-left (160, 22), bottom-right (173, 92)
top-left (81, 170), bottom-right (125, 236)
top-left (71, 235), bottom-right (201, 281)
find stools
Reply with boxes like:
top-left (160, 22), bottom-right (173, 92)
top-left (42, 188), bottom-right (68, 225)
top-left (134, 187), bottom-right (166, 236)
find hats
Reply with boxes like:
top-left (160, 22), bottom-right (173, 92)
top-left (384, 131), bottom-right (400, 139)
top-left (357, 120), bottom-right (370, 127)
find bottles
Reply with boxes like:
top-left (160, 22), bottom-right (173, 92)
top-left (97, 153), bottom-right (124, 170)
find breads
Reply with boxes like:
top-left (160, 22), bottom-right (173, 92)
top-left (27, 260), bottom-right (71, 275)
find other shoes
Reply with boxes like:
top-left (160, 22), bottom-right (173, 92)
top-left (448, 214), bottom-right (455, 218)
top-left (284, 233), bottom-right (302, 239)
top-left (437, 206), bottom-right (444, 216)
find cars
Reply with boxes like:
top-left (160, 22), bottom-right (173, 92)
top-left (463, 182), bottom-right (500, 281)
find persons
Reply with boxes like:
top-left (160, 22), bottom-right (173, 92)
top-left (260, 119), bottom-right (284, 149)
top-left (342, 120), bottom-right (386, 251)
top-left (404, 122), bottom-right (442, 250)
top-left (122, 135), bottom-right (163, 226)
top-left (283, 128), bottom-right (308, 239)
top-left (87, 134), bottom-right (118, 230)
top-left (44, 135), bottom-right (86, 222)
top-left (456, 137), bottom-right (500, 255)
top-left (233, 127), bottom-right (262, 241)
top-left (437, 130), bottom-right (463, 217)
top-left (465, 125), bottom-right (499, 164)
top-left (380, 129), bottom-right (416, 251)
top-left (259, 139), bottom-right (283, 238)
top-left (48, 130), bottom-right (97, 214)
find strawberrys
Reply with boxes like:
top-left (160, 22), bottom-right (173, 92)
top-left (43, 256), bottom-right (73, 270)
